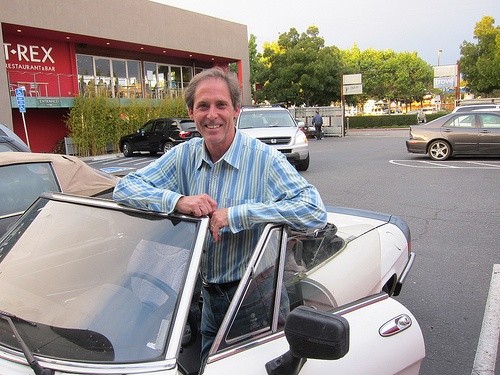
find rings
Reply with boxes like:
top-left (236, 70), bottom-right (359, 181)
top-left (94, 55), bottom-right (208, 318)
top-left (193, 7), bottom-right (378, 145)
top-left (210, 223), bottom-right (214, 227)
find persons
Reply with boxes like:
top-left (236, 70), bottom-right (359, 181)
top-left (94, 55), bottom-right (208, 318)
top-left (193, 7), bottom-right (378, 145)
top-left (113, 68), bottom-right (328, 355)
top-left (312, 111), bottom-right (323, 140)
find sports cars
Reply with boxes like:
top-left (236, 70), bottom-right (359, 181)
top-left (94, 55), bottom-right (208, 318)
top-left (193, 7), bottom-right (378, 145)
top-left (1, 150), bottom-right (426, 375)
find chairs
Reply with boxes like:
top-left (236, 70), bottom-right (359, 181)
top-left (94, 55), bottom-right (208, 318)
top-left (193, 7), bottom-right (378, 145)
top-left (471, 117), bottom-right (484, 128)
top-left (80, 78), bottom-right (178, 100)
top-left (126, 240), bottom-right (188, 305)
top-left (10, 83), bottom-right (40, 97)
top-left (0, 174), bottom-right (44, 215)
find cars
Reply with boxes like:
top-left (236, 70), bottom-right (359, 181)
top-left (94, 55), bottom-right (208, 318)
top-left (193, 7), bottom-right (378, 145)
top-left (118, 117), bottom-right (201, 158)
top-left (406, 103), bottom-right (500, 161)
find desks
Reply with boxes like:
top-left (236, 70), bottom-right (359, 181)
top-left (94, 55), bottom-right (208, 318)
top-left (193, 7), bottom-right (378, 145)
top-left (14, 82), bottom-right (48, 97)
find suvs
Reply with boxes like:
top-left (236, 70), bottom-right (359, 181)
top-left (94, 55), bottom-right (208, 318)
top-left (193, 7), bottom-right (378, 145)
top-left (234, 104), bottom-right (310, 171)
